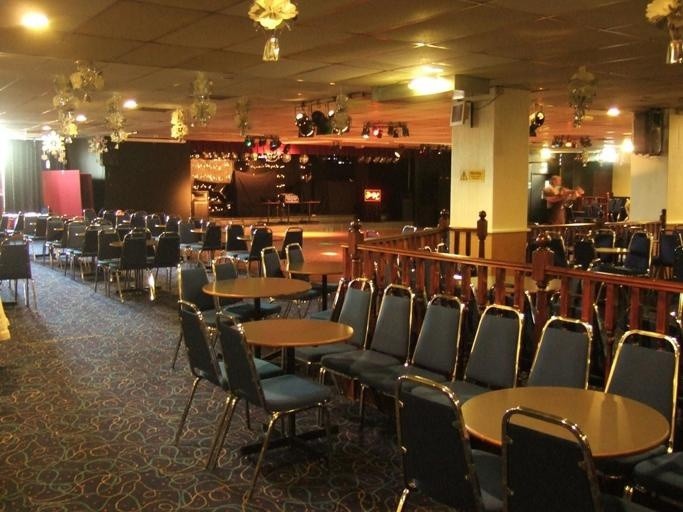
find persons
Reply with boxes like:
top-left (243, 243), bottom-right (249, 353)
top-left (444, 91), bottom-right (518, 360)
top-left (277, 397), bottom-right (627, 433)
top-left (543, 176), bottom-right (561, 206)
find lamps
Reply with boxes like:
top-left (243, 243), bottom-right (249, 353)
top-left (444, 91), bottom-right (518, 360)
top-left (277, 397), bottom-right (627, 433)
top-left (42, 58), bottom-right (128, 169)
top-left (171, 71), bottom-right (447, 166)
top-left (529, 65), bottom-right (598, 168)
top-left (248, 0), bottom-right (299, 64)
top-left (646, 0), bottom-right (683, 64)
